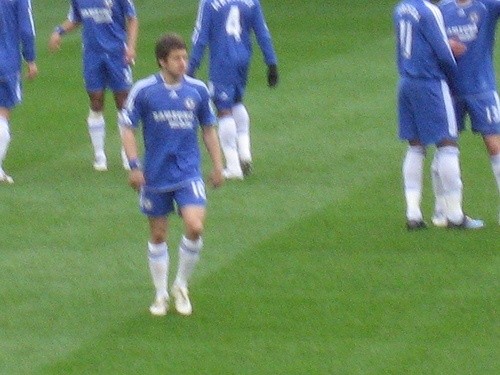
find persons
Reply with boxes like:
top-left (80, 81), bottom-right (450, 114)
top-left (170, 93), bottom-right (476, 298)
top-left (182, 0), bottom-right (277, 181)
top-left (395, 0), bottom-right (484, 232)
top-left (118, 35), bottom-right (224, 315)
top-left (431, 0), bottom-right (500, 227)
top-left (0, 0), bottom-right (38, 181)
top-left (48, 0), bottom-right (144, 172)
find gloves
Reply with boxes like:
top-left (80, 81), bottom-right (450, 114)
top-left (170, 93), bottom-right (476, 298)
top-left (268, 65), bottom-right (278, 85)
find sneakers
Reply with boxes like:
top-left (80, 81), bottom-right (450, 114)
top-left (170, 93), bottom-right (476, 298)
top-left (407, 217), bottom-right (429, 230)
top-left (447, 214), bottom-right (483, 228)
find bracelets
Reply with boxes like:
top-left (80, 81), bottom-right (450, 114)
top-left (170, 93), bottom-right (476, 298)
top-left (55, 26), bottom-right (65, 35)
top-left (128, 160), bottom-right (140, 170)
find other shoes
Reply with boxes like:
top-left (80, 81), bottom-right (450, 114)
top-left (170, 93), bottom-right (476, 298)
top-left (0, 166), bottom-right (15, 185)
top-left (241, 158), bottom-right (253, 176)
top-left (95, 152), bottom-right (107, 171)
top-left (149, 290), bottom-right (169, 315)
top-left (433, 213), bottom-right (447, 227)
top-left (172, 286), bottom-right (193, 315)
top-left (121, 151), bottom-right (140, 170)
top-left (222, 168), bottom-right (243, 180)
